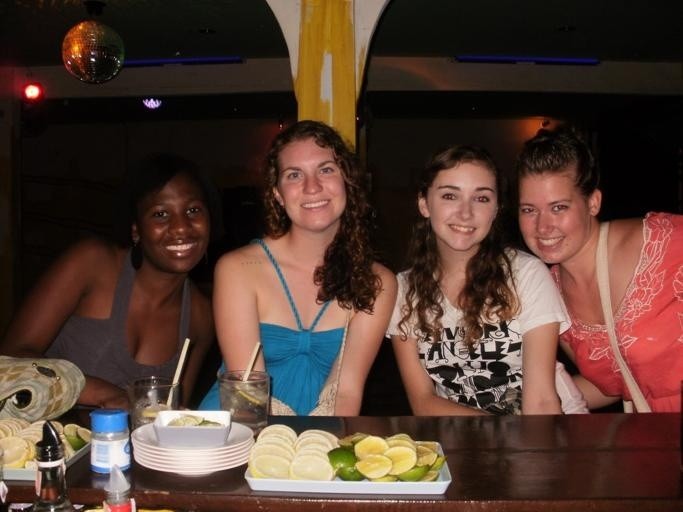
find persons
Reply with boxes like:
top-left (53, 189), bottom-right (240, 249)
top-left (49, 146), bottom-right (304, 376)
top-left (384, 144), bottom-right (592, 418)
top-left (512, 124), bottom-right (683, 414)
top-left (1, 147), bottom-right (216, 412)
top-left (197, 119), bottom-right (398, 417)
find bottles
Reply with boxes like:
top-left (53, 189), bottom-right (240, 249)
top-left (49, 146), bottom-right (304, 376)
top-left (101, 466), bottom-right (136, 512)
top-left (90, 407), bottom-right (131, 475)
top-left (34, 443), bottom-right (77, 512)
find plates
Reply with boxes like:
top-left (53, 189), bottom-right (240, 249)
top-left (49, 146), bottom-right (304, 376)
top-left (128, 423), bottom-right (254, 480)
top-left (245, 439), bottom-right (452, 495)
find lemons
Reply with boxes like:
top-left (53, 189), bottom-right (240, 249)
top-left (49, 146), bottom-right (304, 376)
top-left (0, 419), bottom-right (70, 469)
top-left (143, 405), bottom-right (173, 417)
top-left (248, 424), bottom-right (445, 481)
top-left (166, 416), bottom-right (221, 427)
top-left (64, 421), bottom-right (92, 451)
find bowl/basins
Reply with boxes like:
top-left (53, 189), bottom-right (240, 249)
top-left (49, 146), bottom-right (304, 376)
top-left (154, 409), bottom-right (233, 450)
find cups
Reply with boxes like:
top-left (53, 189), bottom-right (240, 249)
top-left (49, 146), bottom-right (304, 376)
top-left (215, 368), bottom-right (270, 436)
top-left (130, 375), bottom-right (179, 430)
